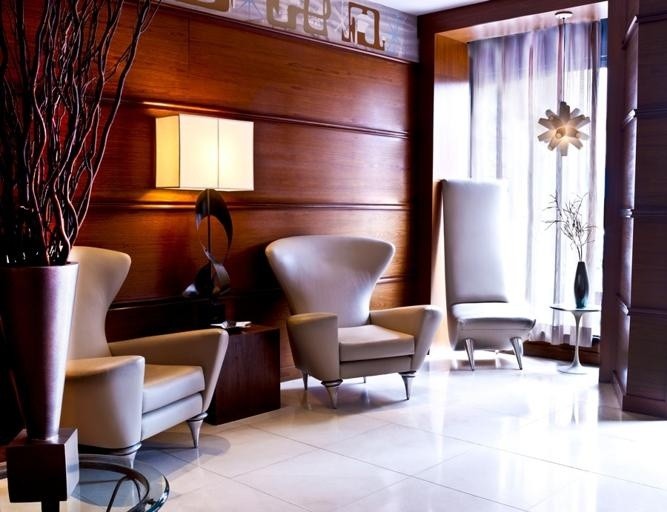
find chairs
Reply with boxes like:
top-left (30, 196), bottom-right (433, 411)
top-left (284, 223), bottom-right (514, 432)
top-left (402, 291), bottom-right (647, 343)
top-left (443, 178), bottom-right (536, 370)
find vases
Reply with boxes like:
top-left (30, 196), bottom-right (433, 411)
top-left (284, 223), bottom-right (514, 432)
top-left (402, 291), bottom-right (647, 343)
top-left (0, 265), bottom-right (70, 502)
top-left (572, 261), bottom-right (591, 311)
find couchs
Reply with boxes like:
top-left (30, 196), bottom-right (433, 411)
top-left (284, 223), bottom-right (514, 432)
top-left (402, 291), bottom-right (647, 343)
top-left (265, 235), bottom-right (440, 410)
top-left (61, 247), bottom-right (229, 470)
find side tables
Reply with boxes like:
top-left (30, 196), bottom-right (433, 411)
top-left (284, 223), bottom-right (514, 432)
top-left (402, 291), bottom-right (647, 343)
top-left (203, 322), bottom-right (282, 425)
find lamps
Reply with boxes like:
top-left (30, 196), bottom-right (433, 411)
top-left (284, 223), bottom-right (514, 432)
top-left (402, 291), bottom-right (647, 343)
top-left (539, 10), bottom-right (590, 158)
top-left (156, 115), bottom-right (254, 192)
